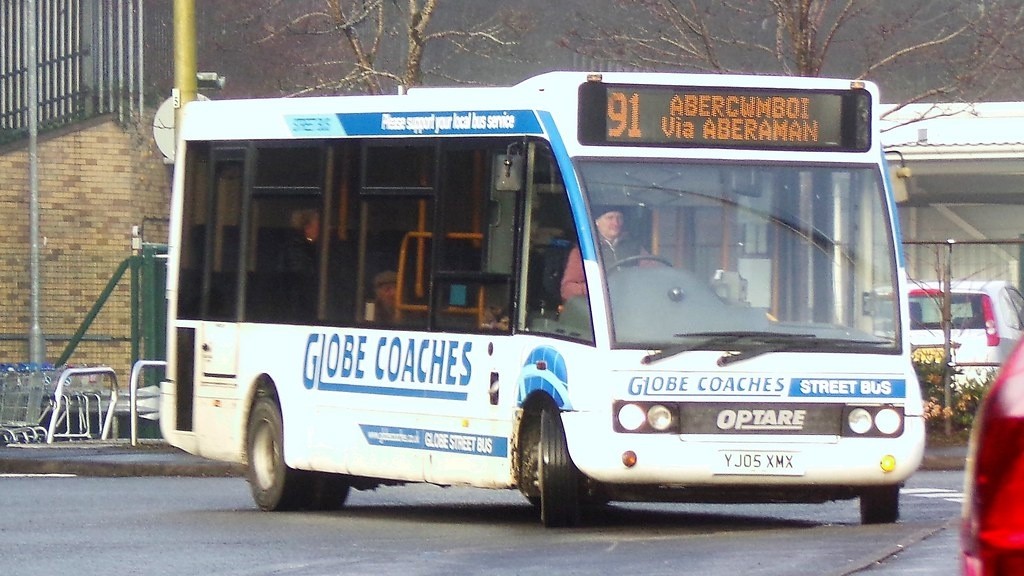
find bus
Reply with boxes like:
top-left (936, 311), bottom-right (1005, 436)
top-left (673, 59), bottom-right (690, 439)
top-left (158, 68), bottom-right (927, 530)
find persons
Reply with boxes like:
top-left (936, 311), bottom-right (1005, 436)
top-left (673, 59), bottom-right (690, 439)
top-left (561, 205), bottom-right (656, 300)
top-left (288, 208), bottom-right (321, 277)
top-left (370, 271), bottom-right (410, 324)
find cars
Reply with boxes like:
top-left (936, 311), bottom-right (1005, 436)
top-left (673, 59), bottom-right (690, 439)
top-left (960, 337), bottom-right (1024, 576)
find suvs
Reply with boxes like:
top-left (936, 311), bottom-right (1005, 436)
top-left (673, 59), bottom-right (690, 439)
top-left (873, 280), bottom-right (1024, 387)
